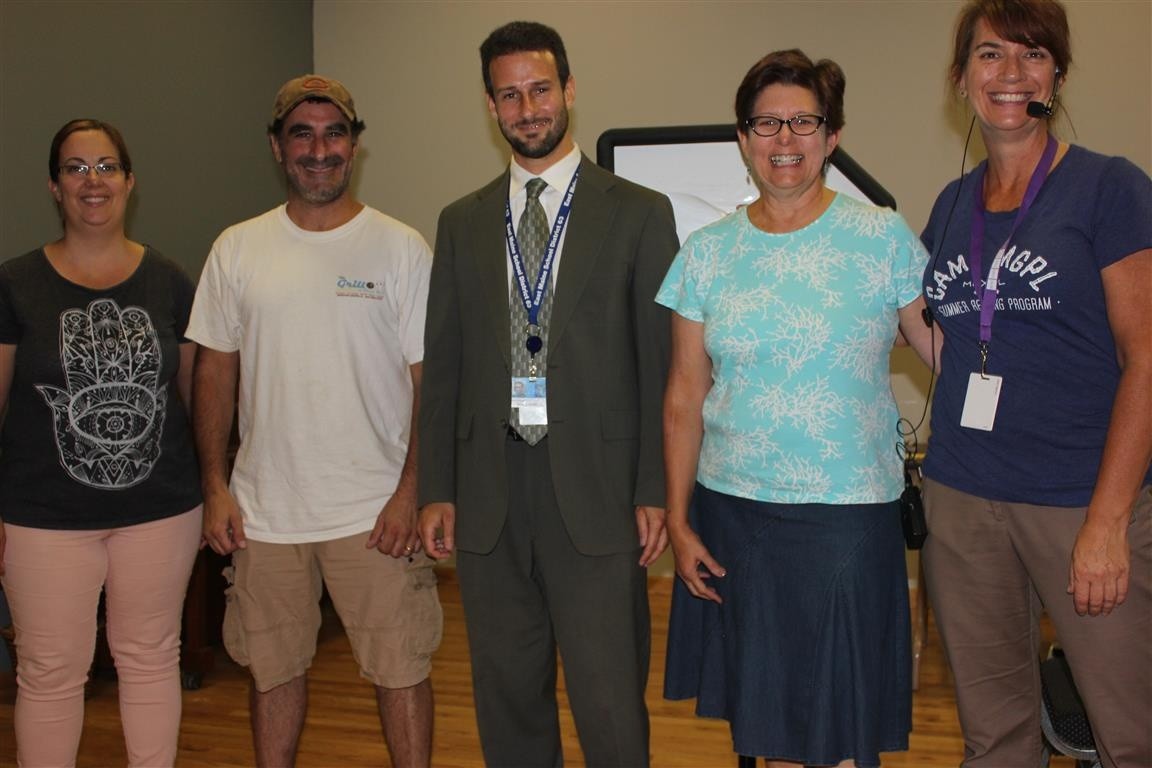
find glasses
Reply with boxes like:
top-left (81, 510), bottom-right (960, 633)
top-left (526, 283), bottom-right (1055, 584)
top-left (747, 114), bottom-right (827, 137)
top-left (58, 162), bottom-right (121, 178)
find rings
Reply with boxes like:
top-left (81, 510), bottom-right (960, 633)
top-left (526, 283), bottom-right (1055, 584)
top-left (406, 544), bottom-right (414, 552)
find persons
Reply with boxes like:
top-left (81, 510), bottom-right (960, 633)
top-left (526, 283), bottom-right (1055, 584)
top-left (513, 382), bottom-right (524, 397)
top-left (184, 74), bottom-right (445, 768)
top-left (415, 22), bottom-right (681, 768)
top-left (655, 50), bottom-right (945, 768)
top-left (896, 0), bottom-right (1152, 768)
top-left (0, 118), bottom-right (204, 768)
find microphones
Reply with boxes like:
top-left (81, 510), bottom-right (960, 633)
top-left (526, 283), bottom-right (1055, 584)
top-left (1027, 73), bottom-right (1059, 118)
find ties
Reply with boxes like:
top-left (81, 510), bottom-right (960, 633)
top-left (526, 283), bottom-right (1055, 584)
top-left (509, 179), bottom-right (551, 446)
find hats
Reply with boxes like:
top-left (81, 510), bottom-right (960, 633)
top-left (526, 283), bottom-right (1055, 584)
top-left (273, 75), bottom-right (357, 126)
